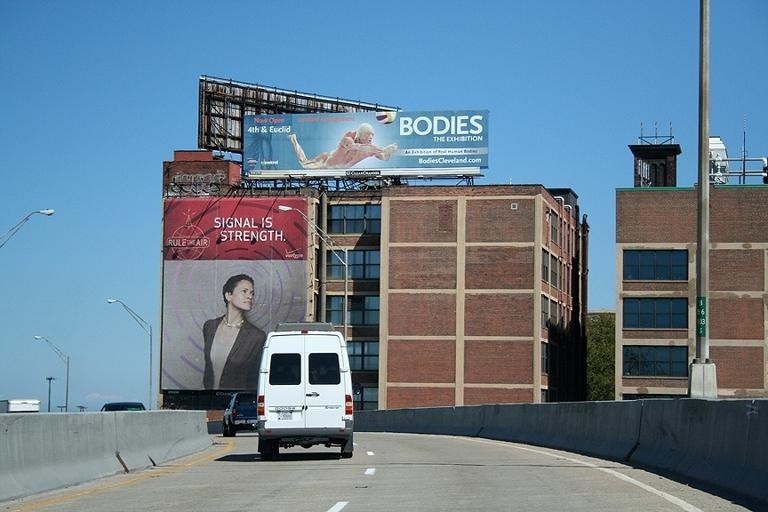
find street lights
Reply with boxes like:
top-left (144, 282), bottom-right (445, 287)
top-left (56, 404), bottom-right (68, 412)
top-left (34, 334), bottom-right (69, 413)
top-left (75, 404), bottom-right (88, 412)
top-left (106, 296), bottom-right (152, 410)
top-left (1, 208), bottom-right (54, 247)
top-left (46, 376), bottom-right (56, 411)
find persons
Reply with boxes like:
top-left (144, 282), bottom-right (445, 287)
top-left (287, 124), bottom-right (399, 170)
top-left (201, 274), bottom-right (270, 389)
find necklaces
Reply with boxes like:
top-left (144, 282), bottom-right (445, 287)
top-left (219, 315), bottom-right (245, 328)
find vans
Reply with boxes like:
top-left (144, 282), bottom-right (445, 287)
top-left (256, 322), bottom-right (362, 459)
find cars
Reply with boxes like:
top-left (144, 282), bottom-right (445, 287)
top-left (101, 402), bottom-right (146, 411)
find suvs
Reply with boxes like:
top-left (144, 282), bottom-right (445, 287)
top-left (221, 390), bottom-right (258, 436)
